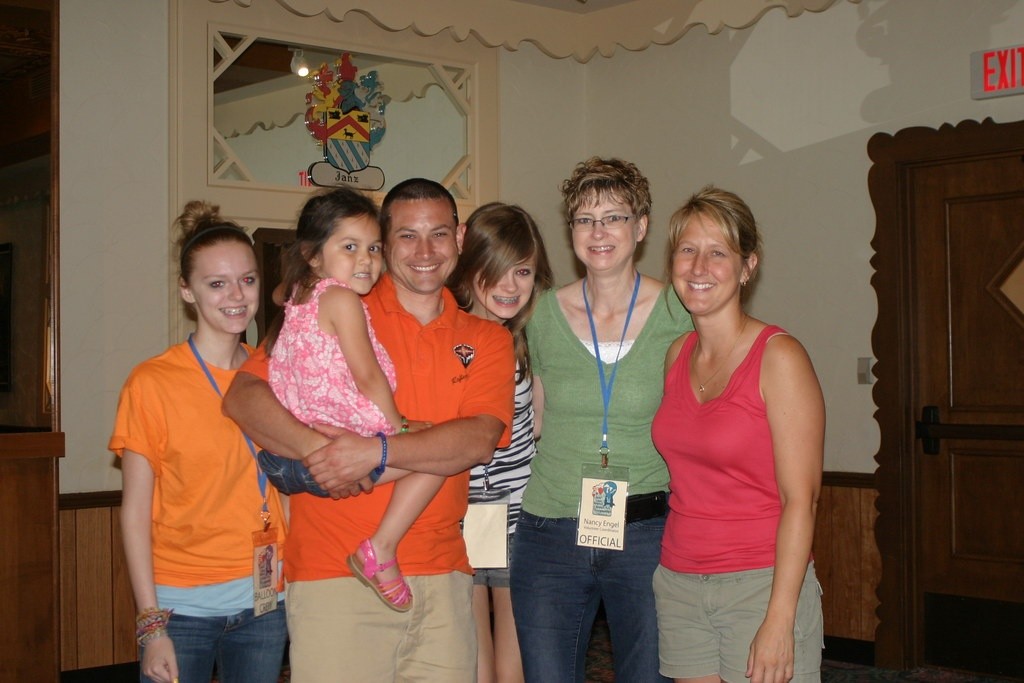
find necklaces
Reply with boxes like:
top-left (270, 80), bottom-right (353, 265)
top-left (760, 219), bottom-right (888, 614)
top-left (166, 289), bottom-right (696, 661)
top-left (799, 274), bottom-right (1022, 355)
top-left (695, 317), bottom-right (747, 392)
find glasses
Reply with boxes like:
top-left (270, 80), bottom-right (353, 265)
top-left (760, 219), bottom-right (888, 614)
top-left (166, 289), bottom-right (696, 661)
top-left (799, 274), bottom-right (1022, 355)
top-left (567, 215), bottom-right (637, 233)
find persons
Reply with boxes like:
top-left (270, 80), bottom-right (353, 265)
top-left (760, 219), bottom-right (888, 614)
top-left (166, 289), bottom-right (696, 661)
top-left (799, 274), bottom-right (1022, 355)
top-left (221, 178), bottom-right (516, 683)
top-left (269, 189), bottom-right (445, 613)
top-left (509, 156), bottom-right (695, 683)
top-left (651, 183), bottom-right (826, 683)
top-left (109, 200), bottom-right (288, 683)
top-left (445, 201), bottom-right (552, 683)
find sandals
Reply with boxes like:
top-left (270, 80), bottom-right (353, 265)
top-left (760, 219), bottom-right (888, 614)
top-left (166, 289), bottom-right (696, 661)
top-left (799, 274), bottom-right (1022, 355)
top-left (347, 538), bottom-right (414, 612)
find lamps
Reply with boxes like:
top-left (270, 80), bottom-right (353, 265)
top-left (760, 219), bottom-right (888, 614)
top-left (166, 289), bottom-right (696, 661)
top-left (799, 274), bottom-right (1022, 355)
top-left (289, 49), bottom-right (307, 77)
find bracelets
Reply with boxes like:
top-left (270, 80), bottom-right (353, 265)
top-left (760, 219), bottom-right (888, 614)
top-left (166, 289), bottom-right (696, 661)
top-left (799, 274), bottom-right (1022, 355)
top-left (375, 433), bottom-right (387, 473)
top-left (135, 607), bottom-right (171, 647)
